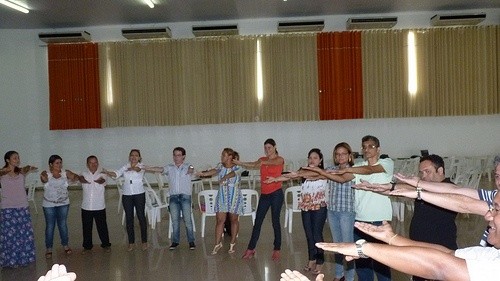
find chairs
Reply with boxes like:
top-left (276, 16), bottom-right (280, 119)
top-left (285, 160), bottom-right (305, 233)
top-left (391, 154), bottom-right (495, 222)
top-left (27, 181), bottom-right (37, 212)
top-left (116, 167), bottom-right (260, 239)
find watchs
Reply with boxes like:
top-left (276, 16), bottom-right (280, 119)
top-left (356, 239), bottom-right (369, 258)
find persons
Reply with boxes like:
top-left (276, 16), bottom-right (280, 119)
top-left (389, 158), bottom-right (500, 247)
top-left (196, 148), bottom-right (243, 254)
top-left (302, 137), bottom-right (395, 281)
top-left (262, 143), bottom-right (356, 281)
top-left (350, 154), bottom-right (459, 281)
top-left (315, 189), bottom-right (500, 281)
top-left (78, 155), bottom-right (111, 255)
top-left (143, 147), bottom-right (195, 249)
top-left (233, 139), bottom-right (285, 261)
top-left (41, 155), bottom-right (74, 258)
top-left (101, 149), bottom-right (148, 251)
top-left (0, 151), bottom-right (39, 267)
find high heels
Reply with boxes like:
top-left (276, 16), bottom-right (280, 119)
top-left (240, 248), bottom-right (256, 260)
top-left (303, 258), bottom-right (316, 272)
top-left (209, 241), bottom-right (223, 255)
top-left (271, 250), bottom-right (281, 260)
top-left (312, 263), bottom-right (325, 276)
top-left (228, 243), bottom-right (236, 253)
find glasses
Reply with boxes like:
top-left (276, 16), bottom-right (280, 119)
top-left (360, 144), bottom-right (377, 150)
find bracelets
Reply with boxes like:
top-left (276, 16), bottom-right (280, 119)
top-left (390, 182), bottom-right (396, 191)
top-left (417, 179), bottom-right (421, 187)
top-left (390, 233), bottom-right (399, 245)
top-left (417, 189), bottom-right (421, 199)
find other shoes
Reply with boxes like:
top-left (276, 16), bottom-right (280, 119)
top-left (141, 242), bottom-right (148, 250)
top-left (64, 247), bottom-right (72, 255)
top-left (45, 250), bottom-right (52, 258)
top-left (103, 247), bottom-right (112, 256)
top-left (127, 244), bottom-right (136, 252)
top-left (81, 248), bottom-right (91, 255)
top-left (332, 276), bottom-right (345, 281)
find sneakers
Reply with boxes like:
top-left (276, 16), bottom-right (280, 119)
top-left (188, 241), bottom-right (196, 250)
top-left (169, 242), bottom-right (180, 250)
